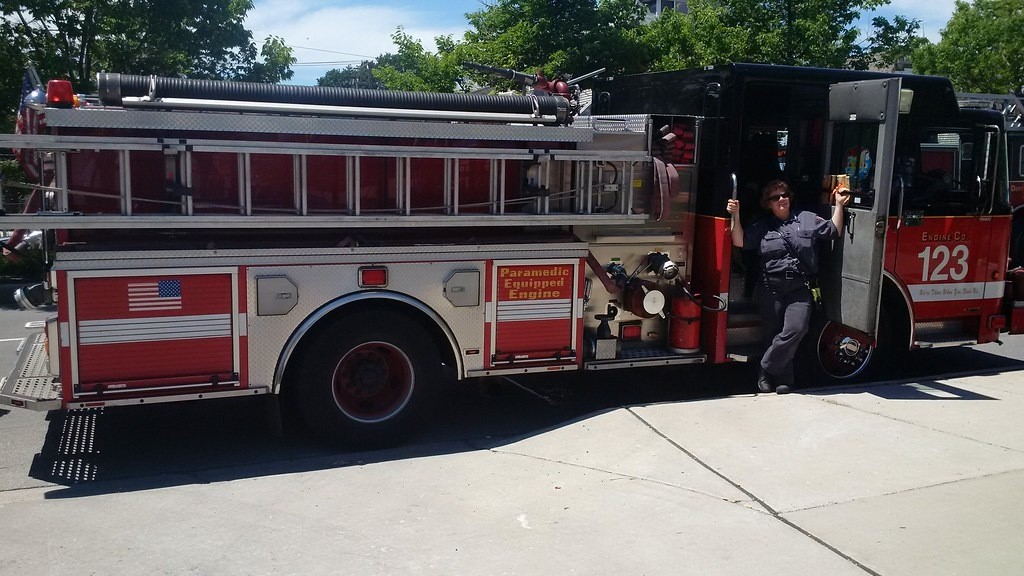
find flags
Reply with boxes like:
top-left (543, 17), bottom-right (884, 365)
top-left (13, 69), bottom-right (46, 179)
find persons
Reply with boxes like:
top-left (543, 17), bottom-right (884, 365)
top-left (727, 179), bottom-right (851, 394)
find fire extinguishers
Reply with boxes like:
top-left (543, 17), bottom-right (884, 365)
top-left (670, 276), bottom-right (703, 355)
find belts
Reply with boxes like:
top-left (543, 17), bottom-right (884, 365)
top-left (768, 270), bottom-right (800, 280)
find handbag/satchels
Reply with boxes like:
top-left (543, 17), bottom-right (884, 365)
top-left (811, 285), bottom-right (826, 328)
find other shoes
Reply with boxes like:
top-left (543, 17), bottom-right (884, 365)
top-left (757, 366), bottom-right (771, 393)
top-left (776, 384), bottom-right (789, 394)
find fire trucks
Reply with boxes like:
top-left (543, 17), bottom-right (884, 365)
top-left (0, 55), bottom-right (1024, 442)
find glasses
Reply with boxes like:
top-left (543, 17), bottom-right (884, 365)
top-left (767, 192), bottom-right (789, 201)
